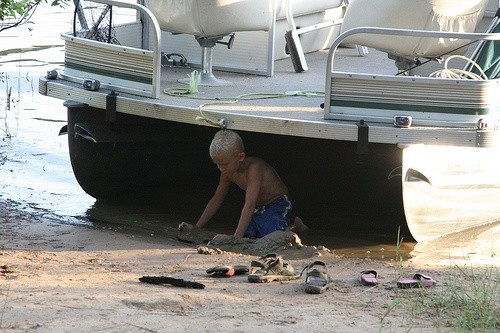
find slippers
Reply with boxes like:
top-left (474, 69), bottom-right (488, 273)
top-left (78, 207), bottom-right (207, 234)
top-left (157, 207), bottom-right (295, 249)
top-left (396, 272), bottom-right (439, 287)
top-left (204, 264), bottom-right (250, 276)
top-left (358, 268), bottom-right (380, 285)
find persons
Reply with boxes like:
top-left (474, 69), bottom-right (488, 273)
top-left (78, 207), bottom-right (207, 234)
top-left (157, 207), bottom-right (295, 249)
top-left (170, 129), bottom-right (309, 245)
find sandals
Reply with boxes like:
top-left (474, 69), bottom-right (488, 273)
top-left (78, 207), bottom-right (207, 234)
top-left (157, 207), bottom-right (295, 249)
top-left (299, 260), bottom-right (335, 293)
top-left (248, 252), bottom-right (302, 283)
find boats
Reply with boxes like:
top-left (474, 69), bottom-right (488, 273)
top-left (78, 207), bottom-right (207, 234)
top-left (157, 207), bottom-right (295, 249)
top-left (39, 0), bottom-right (500, 246)
